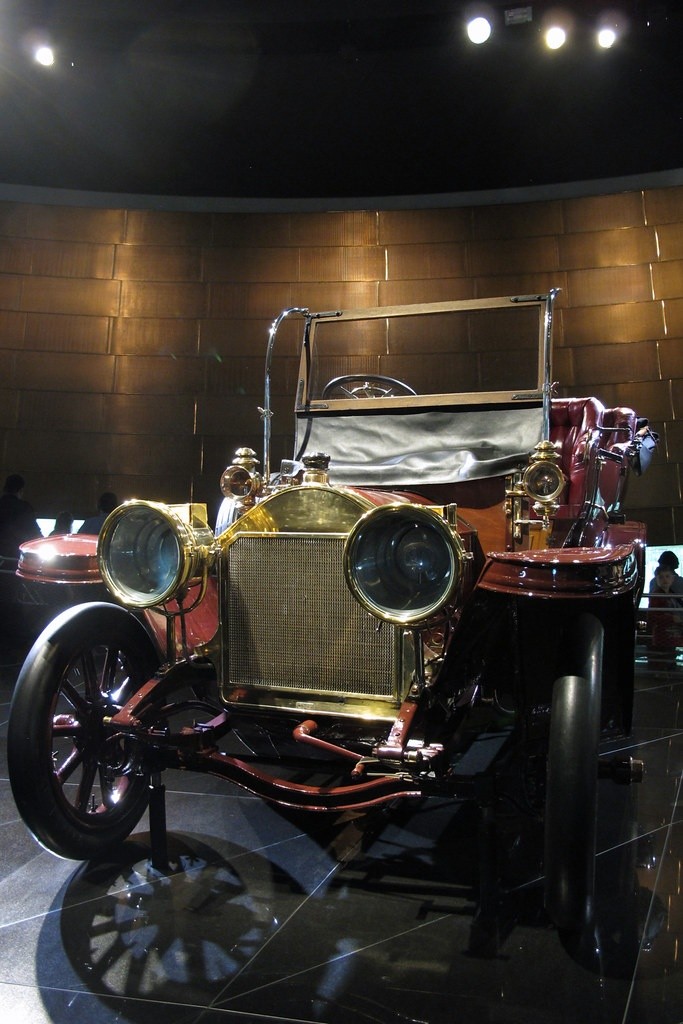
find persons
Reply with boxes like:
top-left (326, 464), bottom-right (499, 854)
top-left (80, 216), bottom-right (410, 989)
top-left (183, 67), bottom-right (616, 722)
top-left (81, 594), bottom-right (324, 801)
top-left (49, 512), bottom-right (73, 536)
top-left (77, 492), bottom-right (119, 533)
top-left (650, 550), bottom-right (683, 600)
top-left (648, 565), bottom-right (683, 670)
top-left (0, 474), bottom-right (43, 569)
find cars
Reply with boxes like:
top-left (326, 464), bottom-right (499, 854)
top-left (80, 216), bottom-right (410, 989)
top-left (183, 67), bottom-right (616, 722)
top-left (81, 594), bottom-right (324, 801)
top-left (0, 285), bottom-right (665, 932)
